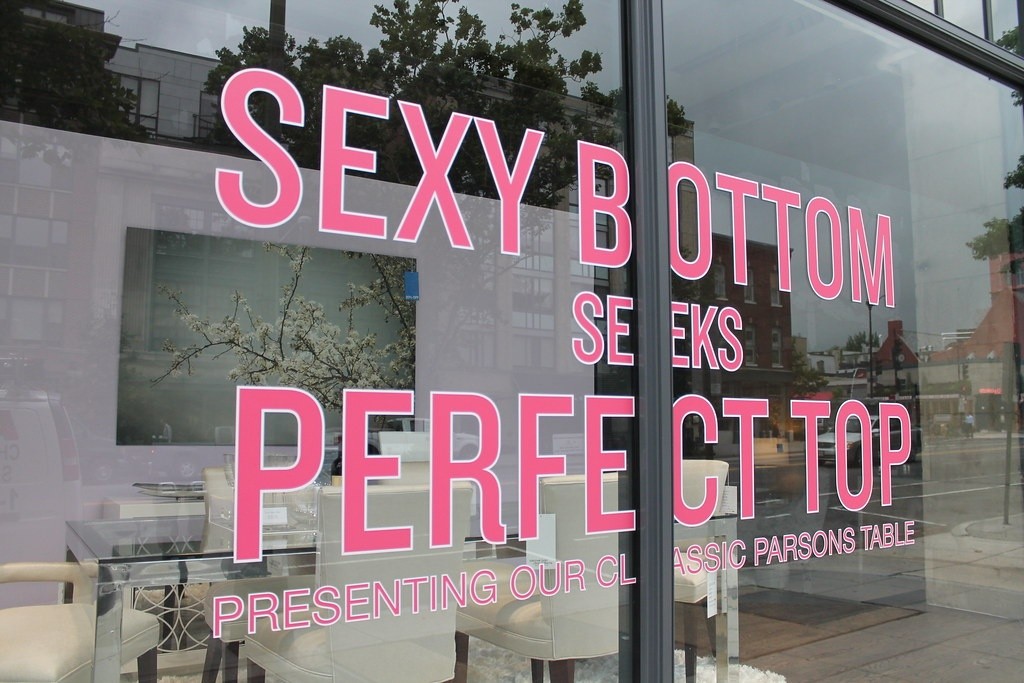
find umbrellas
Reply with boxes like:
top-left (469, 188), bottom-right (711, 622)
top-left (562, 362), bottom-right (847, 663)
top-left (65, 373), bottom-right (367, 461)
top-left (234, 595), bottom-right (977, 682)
top-left (929, 286), bottom-right (1024, 524)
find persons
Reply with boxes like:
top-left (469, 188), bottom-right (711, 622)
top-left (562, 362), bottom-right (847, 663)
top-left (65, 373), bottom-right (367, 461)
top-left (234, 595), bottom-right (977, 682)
top-left (964, 411), bottom-right (973, 439)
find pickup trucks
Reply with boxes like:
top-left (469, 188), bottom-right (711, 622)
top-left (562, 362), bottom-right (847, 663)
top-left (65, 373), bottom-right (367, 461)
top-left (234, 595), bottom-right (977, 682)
top-left (821, 416), bottom-right (923, 468)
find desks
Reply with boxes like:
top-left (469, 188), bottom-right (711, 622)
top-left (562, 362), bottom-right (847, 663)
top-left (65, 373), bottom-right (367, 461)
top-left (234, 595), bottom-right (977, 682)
top-left (62, 500), bottom-right (739, 683)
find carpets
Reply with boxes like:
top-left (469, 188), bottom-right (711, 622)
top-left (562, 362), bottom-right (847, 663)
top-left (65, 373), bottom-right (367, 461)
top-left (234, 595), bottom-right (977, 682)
top-left (157, 635), bottom-right (787, 683)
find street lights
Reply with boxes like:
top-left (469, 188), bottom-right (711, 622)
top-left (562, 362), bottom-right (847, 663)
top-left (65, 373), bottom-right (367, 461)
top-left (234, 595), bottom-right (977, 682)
top-left (866, 294), bottom-right (886, 397)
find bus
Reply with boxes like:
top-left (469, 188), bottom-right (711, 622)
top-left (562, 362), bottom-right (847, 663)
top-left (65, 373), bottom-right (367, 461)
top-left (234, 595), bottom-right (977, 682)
top-left (867, 393), bottom-right (976, 438)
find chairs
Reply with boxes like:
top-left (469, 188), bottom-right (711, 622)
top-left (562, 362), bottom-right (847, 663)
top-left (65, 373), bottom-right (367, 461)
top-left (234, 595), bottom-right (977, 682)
top-left (200, 466), bottom-right (319, 683)
top-left (672, 458), bottom-right (730, 683)
top-left (245, 479), bottom-right (476, 683)
top-left (0, 562), bottom-right (161, 683)
top-left (454, 471), bottom-right (619, 683)
